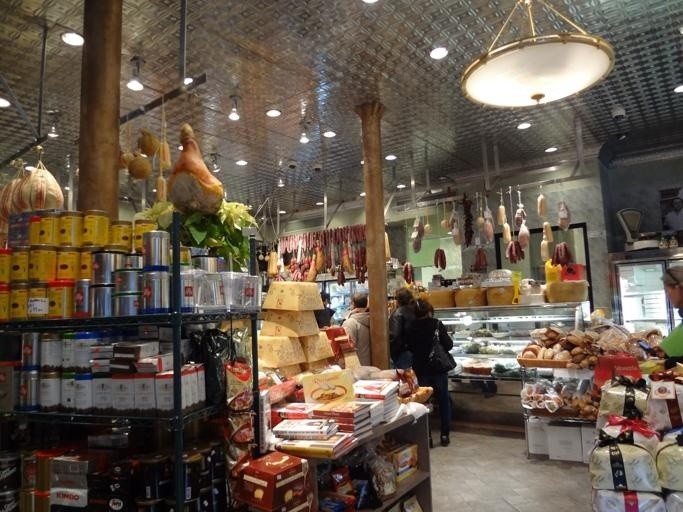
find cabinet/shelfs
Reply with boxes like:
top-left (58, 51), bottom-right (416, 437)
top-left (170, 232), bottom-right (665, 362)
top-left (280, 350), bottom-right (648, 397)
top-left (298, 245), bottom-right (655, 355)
top-left (0, 212), bottom-right (260, 511)
top-left (425, 302), bottom-right (590, 437)
top-left (517, 358), bottom-right (597, 464)
top-left (309, 407), bottom-right (431, 512)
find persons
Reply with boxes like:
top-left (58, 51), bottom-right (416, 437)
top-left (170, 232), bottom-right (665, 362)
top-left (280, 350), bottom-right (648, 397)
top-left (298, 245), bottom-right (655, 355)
top-left (655, 263), bottom-right (682, 362)
top-left (406, 296), bottom-right (457, 447)
top-left (312, 291), bottom-right (338, 328)
top-left (664, 196), bottom-right (683, 230)
top-left (387, 286), bottom-right (415, 367)
top-left (341, 291), bottom-right (371, 366)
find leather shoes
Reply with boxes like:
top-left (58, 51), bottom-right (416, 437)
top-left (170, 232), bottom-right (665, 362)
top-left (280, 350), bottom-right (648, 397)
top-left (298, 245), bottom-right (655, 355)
top-left (429, 438), bottom-right (433, 448)
top-left (441, 432), bottom-right (449, 446)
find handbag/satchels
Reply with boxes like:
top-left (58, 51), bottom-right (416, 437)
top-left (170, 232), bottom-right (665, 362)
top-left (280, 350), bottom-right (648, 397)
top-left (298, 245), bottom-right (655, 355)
top-left (426, 320), bottom-right (456, 375)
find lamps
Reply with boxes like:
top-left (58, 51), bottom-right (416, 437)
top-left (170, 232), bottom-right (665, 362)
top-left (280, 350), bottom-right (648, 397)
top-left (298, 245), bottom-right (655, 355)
top-left (228, 92), bottom-right (242, 122)
top-left (45, 117), bottom-right (61, 139)
top-left (208, 153), bottom-right (224, 174)
top-left (298, 120), bottom-right (311, 144)
top-left (125, 52), bottom-right (145, 91)
top-left (458, 0), bottom-right (618, 110)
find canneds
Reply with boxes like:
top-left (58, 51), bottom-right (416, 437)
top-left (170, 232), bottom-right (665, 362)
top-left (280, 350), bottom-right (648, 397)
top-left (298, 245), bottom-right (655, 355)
top-left (0, 208), bottom-right (261, 323)
top-left (1, 329), bottom-right (127, 413)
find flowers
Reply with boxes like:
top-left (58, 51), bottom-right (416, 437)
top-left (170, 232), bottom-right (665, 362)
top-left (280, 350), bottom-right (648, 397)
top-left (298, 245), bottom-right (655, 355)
top-left (139, 192), bottom-right (258, 273)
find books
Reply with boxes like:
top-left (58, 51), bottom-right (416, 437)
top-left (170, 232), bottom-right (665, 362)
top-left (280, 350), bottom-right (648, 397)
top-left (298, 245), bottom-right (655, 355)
top-left (271, 379), bottom-right (401, 462)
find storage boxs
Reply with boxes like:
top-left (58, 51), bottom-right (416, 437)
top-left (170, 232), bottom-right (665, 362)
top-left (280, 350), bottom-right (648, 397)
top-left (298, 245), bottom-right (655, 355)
top-left (525, 416), bottom-right (599, 463)
